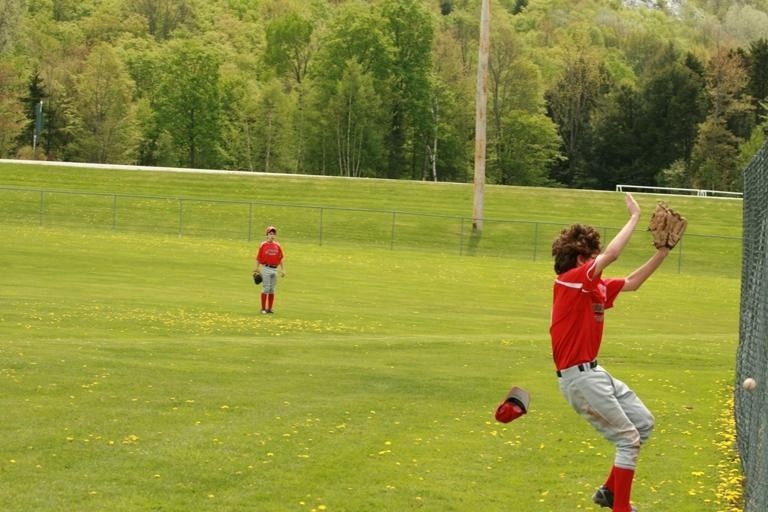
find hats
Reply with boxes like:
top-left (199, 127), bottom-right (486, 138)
top-left (266, 226), bottom-right (276, 233)
top-left (495, 387), bottom-right (531, 423)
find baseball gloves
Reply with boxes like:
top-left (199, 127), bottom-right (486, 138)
top-left (253, 270), bottom-right (262, 284)
top-left (648, 199), bottom-right (687, 250)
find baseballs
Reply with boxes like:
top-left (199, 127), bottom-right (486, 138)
top-left (743, 378), bottom-right (755, 391)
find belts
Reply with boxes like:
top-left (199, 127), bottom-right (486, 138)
top-left (265, 264), bottom-right (277, 268)
top-left (556, 360), bottom-right (598, 378)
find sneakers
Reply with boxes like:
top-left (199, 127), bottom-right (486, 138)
top-left (592, 485), bottom-right (637, 512)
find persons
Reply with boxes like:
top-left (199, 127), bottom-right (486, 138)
top-left (253, 224), bottom-right (286, 316)
top-left (549, 190), bottom-right (688, 512)
